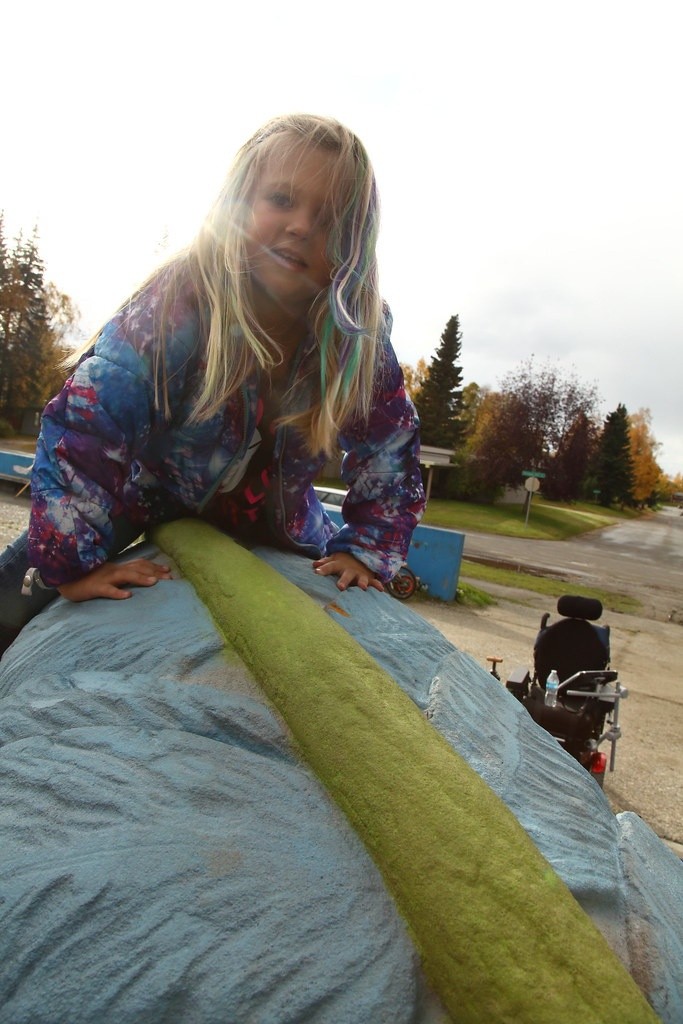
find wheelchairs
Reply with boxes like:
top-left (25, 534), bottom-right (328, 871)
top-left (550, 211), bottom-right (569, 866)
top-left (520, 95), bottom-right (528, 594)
top-left (481, 592), bottom-right (628, 795)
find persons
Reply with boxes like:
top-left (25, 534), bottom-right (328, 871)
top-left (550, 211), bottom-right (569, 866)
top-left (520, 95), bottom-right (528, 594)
top-left (0, 117), bottom-right (426, 654)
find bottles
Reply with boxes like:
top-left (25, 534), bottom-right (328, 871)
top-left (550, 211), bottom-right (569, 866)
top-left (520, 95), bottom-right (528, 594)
top-left (544, 669), bottom-right (559, 708)
